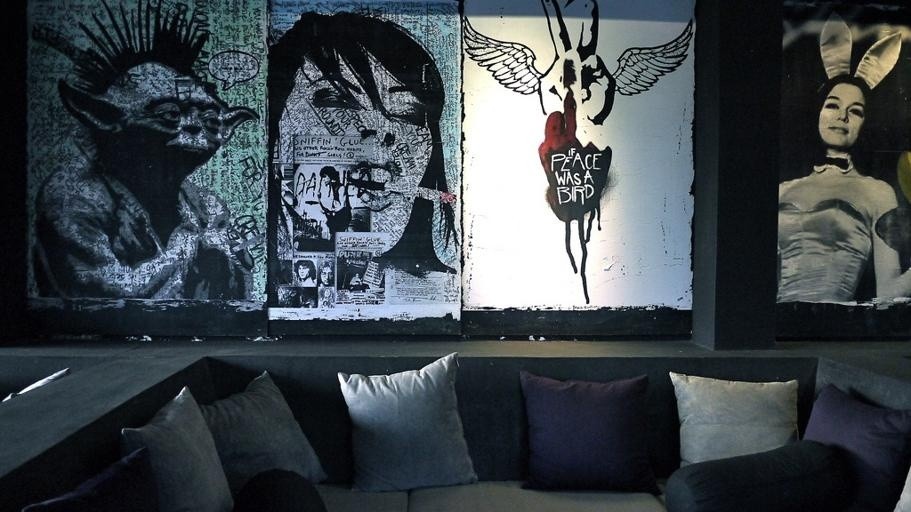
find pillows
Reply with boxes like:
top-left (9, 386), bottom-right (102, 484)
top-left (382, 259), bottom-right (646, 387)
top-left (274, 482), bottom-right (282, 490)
top-left (121, 386), bottom-right (233, 511)
top-left (520, 370), bottom-right (662, 494)
top-left (669, 372), bottom-right (798, 468)
top-left (201, 370), bottom-right (328, 482)
top-left (665, 439), bottom-right (853, 512)
top-left (802, 384), bottom-right (911, 511)
top-left (21, 446), bottom-right (155, 511)
top-left (337, 352), bottom-right (477, 492)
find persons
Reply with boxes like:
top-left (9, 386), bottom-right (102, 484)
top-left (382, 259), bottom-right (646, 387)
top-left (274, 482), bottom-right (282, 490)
top-left (777, 73), bottom-right (911, 302)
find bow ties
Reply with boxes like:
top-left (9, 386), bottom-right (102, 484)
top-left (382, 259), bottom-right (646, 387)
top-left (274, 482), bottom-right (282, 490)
top-left (812, 157), bottom-right (849, 170)
